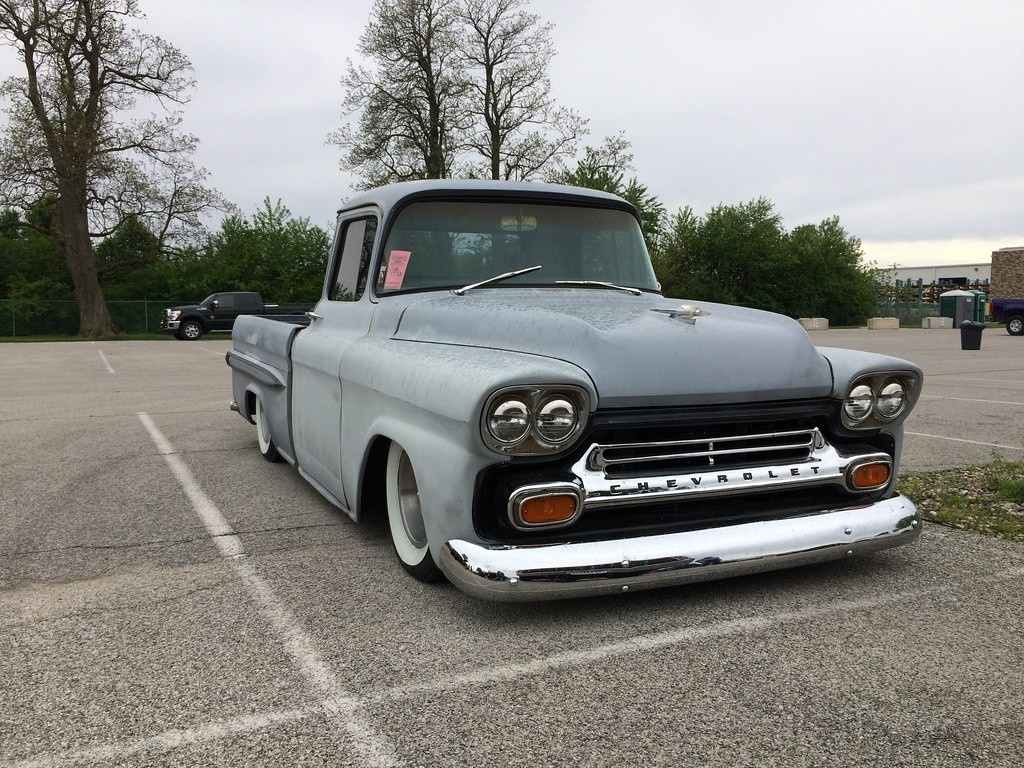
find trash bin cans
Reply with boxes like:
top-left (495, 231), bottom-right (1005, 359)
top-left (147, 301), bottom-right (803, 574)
top-left (959, 320), bottom-right (986, 350)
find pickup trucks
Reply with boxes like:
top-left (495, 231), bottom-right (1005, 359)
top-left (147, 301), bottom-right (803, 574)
top-left (989, 296), bottom-right (1024, 337)
top-left (159, 291), bottom-right (310, 341)
top-left (226, 175), bottom-right (925, 606)
